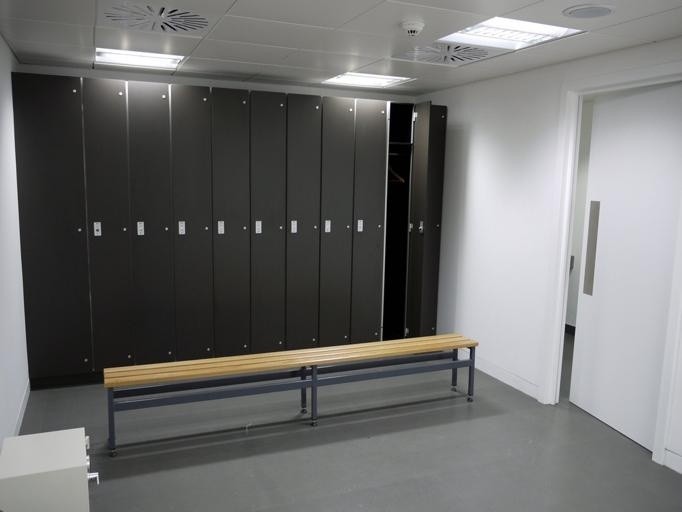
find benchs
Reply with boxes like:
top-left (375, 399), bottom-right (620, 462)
top-left (102, 332), bottom-right (478, 457)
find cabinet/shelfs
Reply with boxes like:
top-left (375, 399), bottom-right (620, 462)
top-left (0, 426), bottom-right (100, 512)
top-left (11, 71), bottom-right (448, 391)
